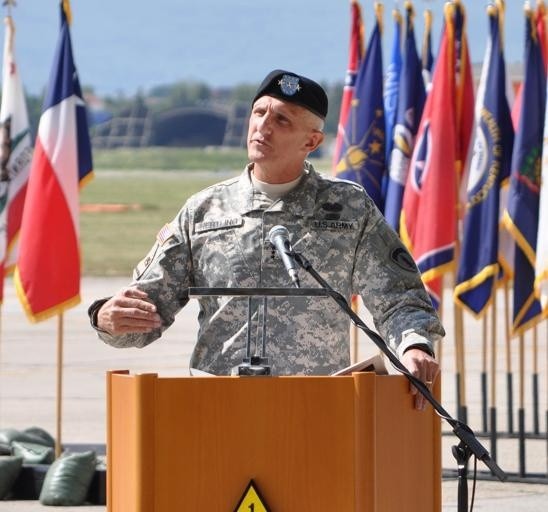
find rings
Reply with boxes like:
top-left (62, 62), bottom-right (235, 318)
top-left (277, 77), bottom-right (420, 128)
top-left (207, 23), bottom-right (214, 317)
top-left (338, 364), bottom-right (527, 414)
top-left (425, 381), bottom-right (434, 385)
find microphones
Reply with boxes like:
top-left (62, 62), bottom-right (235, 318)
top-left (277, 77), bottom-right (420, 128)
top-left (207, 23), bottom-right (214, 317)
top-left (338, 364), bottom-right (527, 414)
top-left (269, 225), bottom-right (300, 288)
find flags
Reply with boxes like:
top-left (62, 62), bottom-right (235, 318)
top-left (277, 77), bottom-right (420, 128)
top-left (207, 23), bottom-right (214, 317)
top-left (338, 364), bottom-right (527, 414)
top-left (12, 0), bottom-right (95, 325)
top-left (328, 0), bottom-right (548, 339)
top-left (0, 16), bottom-right (33, 307)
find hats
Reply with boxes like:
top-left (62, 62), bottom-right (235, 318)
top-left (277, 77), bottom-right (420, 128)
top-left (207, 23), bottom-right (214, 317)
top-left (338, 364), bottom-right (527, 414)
top-left (251, 68), bottom-right (329, 121)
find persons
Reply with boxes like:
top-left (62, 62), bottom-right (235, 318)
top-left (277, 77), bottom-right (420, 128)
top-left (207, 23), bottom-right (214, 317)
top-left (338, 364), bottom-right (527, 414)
top-left (84, 68), bottom-right (446, 413)
